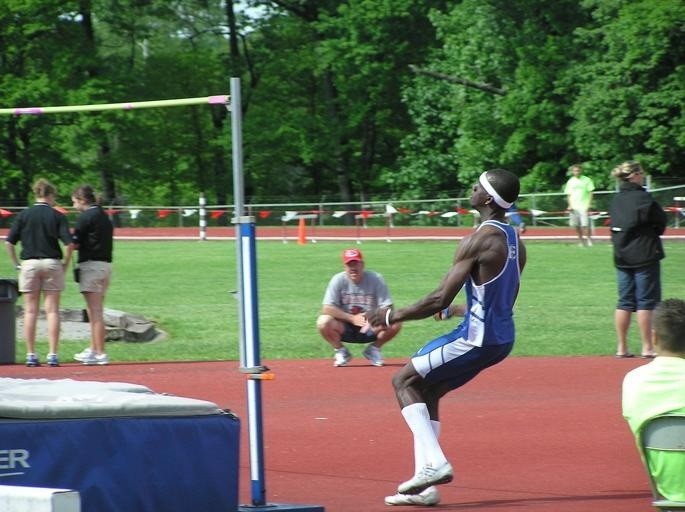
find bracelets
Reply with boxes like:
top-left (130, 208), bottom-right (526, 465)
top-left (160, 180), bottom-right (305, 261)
top-left (385, 308), bottom-right (391, 328)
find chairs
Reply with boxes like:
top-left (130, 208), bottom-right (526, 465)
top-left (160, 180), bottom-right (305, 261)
top-left (637, 414), bottom-right (685, 512)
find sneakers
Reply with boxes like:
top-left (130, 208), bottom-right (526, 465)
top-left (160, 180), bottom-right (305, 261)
top-left (398, 461), bottom-right (453, 493)
top-left (25, 349), bottom-right (107, 366)
top-left (384, 490), bottom-right (439, 505)
top-left (364, 344), bottom-right (383, 367)
top-left (334, 346), bottom-right (351, 366)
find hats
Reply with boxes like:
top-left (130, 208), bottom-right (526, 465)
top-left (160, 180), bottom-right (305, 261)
top-left (342, 248), bottom-right (362, 264)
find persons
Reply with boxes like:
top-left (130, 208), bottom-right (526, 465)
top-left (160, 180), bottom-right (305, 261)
top-left (507, 202), bottom-right (525, 235)
top-left (563, 166), bottom-right (597, 251)
top-left (617, 298), bottom-right (685, 503)
top-left (362, 167), bottom-right (528, 508)
top-left (606, 159), bottom-right (669, 359)
top-left (315, 245), bottom-right (402, 367)
top-left (4, 177), bottom-right (76, 366)
top-left (70, 183), bottom-right (115, 368)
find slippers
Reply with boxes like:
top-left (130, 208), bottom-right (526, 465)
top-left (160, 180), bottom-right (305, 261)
top-left (615, 352), bottom-right (633, 358)
top-left (641, 353), bottom-right (656, 359)
